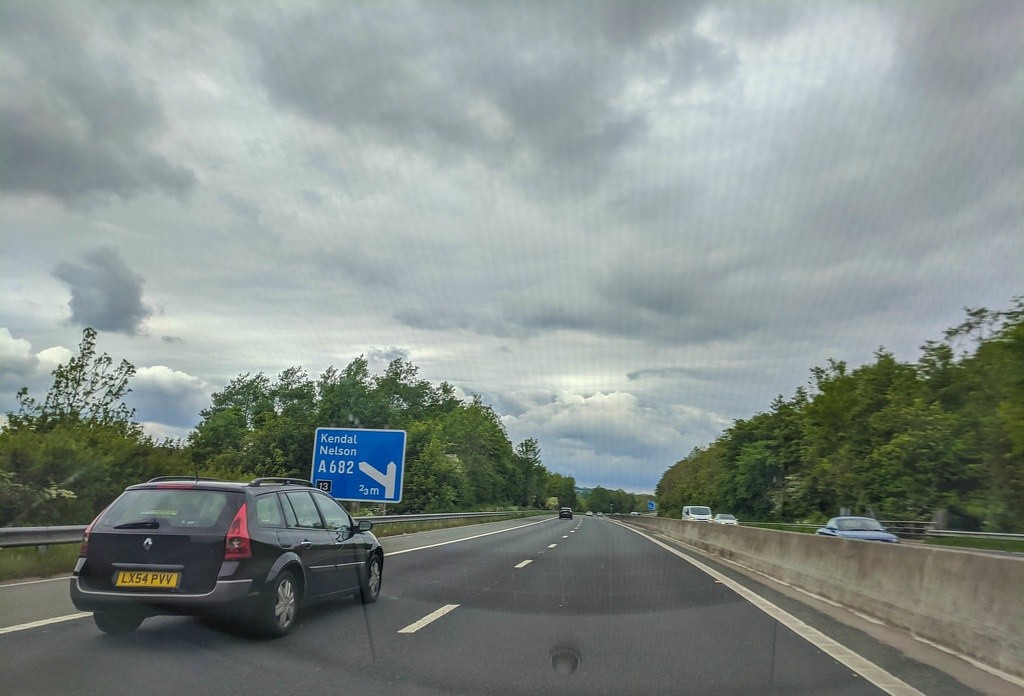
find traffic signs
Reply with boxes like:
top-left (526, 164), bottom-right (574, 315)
top-left (310, 426), bottom-right (407, 504)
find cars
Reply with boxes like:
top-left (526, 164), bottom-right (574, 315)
top-left (682, 503), bottom-right (712, 522)
top-left (713, 513), bottom-right (739, 525)
top-left (67, 475), bottom-right (385, 634)
top-left (815, 516), bottom-right (899, 543)
top-left (559, 506), bottom-right (572, 520)
top-left (586, 510), bottom-right (641, 517)
